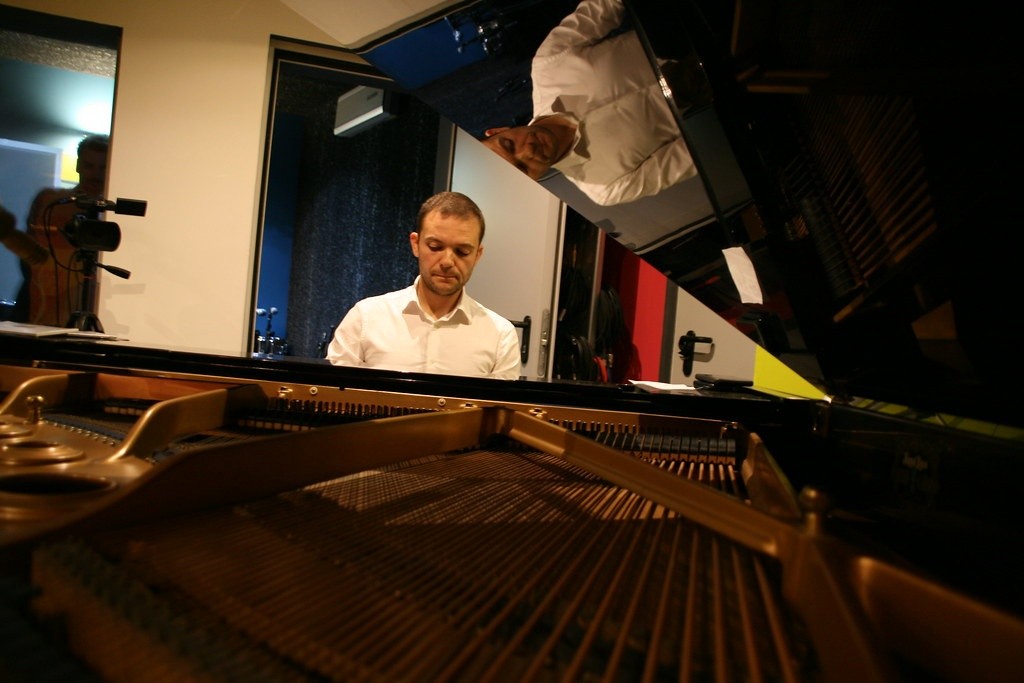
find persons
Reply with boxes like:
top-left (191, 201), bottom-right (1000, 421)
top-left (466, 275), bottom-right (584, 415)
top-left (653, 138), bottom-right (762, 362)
top-left (326, 192), bottom-right (521, 381)
top-left (476, 0), bottom-right (699, 206)
top-left (15, 133), bottom-right (110, 329)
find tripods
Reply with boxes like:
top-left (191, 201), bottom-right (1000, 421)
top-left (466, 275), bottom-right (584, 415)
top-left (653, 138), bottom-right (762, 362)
top-left (64, 248), bottom-right (131, 334)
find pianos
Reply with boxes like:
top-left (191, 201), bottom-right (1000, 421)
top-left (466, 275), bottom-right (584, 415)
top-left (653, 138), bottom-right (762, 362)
top-left (1, 1), bottom-right (1023, 683)
